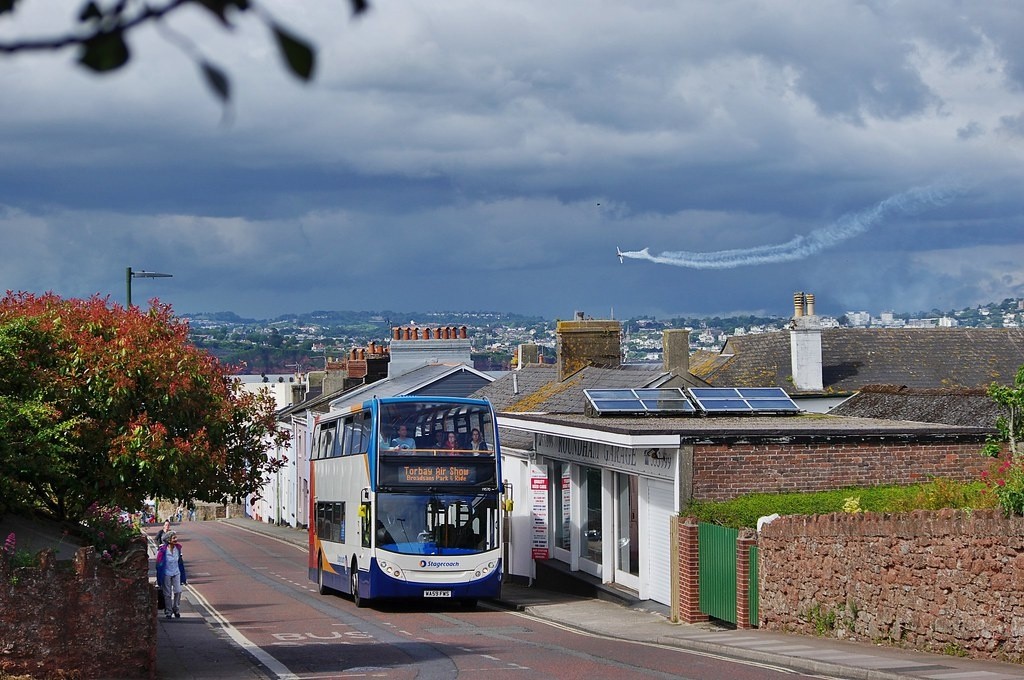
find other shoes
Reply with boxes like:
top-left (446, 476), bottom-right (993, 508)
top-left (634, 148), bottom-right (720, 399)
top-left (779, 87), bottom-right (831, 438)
top-left (174, 610), bottom-right (181, 619)
top-left (166, 614), bottom-right (171, 619)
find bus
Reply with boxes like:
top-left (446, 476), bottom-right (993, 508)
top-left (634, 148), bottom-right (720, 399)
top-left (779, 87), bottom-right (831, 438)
top-left (309, 393), bottom-right (516, 608)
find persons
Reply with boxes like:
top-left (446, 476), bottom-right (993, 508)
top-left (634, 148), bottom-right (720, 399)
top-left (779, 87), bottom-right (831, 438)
top-left (154, 519), bottom-right (172, 551)
top-left (392, 424), bottom-right (416, 454)
top-left (462, 428), bottom-right (489, 456)
top-left (432, 431), bottom-right (443, 449)
top-left (156, 531), bottom-right (187, 618)
top-left (176, 501), bottom-right (185, 521)
top-left (379, 423), bottom-right (399, 452)
top-left (440, 432), bottom-right (460, 456)
top-left (188, 500), bottom-right (197, 521)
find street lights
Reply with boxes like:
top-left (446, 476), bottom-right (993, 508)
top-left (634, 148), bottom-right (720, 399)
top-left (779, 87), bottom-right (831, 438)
top-left (125, 267), bottom-right (173, 310)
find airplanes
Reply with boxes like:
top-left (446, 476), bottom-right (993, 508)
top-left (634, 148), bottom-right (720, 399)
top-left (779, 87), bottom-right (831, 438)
top-left (616, 246), bottom-right (624, 263)
